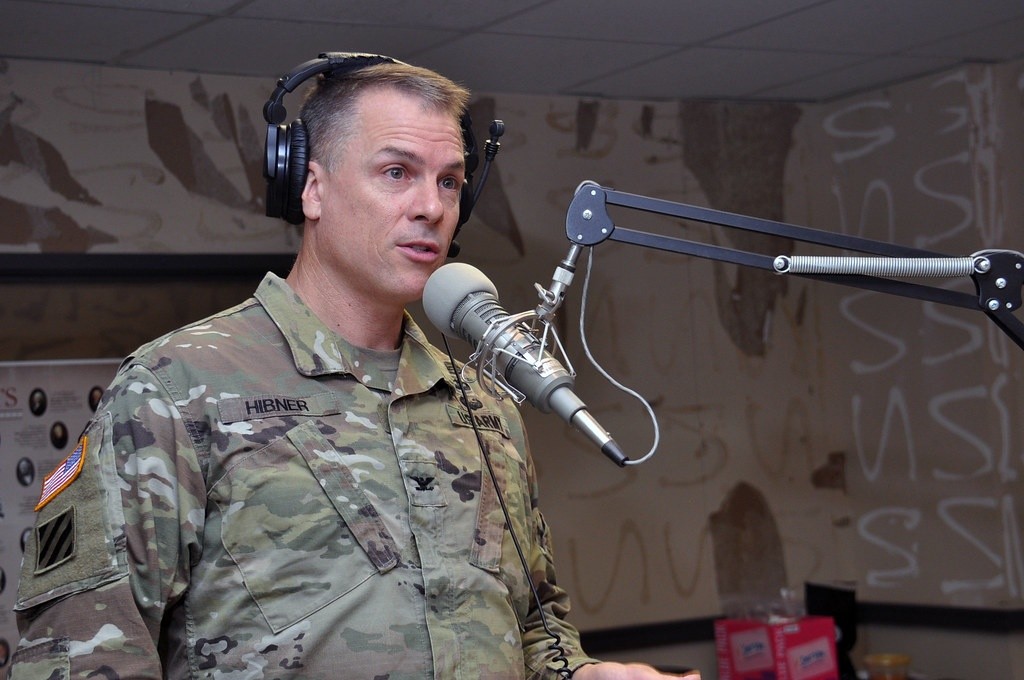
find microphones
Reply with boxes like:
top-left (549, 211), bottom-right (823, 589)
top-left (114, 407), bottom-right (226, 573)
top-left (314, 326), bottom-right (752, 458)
top-left (422, 262), bottom-right (632, 470)
top-left (474, 118), bottom-right (505, 205)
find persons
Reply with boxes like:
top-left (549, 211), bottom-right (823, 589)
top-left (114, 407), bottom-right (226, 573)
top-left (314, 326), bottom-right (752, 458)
top-left (15, 390), bottom-right (109, 549)
top-left (6, 62), bottom-right (702, 680)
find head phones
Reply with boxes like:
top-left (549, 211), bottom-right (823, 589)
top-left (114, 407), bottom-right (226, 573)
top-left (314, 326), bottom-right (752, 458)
top-left (262, 51), bottom-right (478, 260)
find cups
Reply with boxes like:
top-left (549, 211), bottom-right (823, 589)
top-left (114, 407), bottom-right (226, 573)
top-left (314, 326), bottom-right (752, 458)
top-left (863, 653), bottom-right (911, 680)
